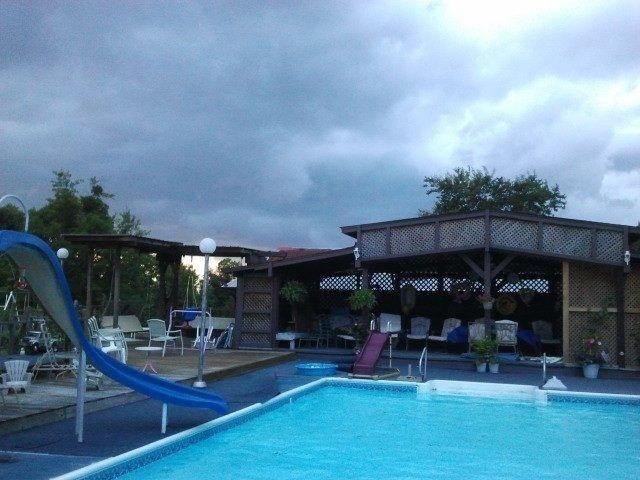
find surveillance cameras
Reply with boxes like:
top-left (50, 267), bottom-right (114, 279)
top-left (625, 251), bottom-right (630, 259)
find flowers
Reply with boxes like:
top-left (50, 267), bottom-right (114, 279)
top-left (576, 329), bottom-right (611, 362)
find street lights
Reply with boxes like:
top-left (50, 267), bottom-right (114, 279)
top-left (55, 247), bottom-right (70, 267)
top-left (190, 238), bottom-right (218, 389)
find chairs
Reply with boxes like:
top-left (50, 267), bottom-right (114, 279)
top-left (274, 305), bottom-right (562, 376)
top-left (1, 308), bottom-right (234, 399)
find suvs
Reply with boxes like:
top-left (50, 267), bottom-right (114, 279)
top-left (19, 331), bottom-right (59, 352)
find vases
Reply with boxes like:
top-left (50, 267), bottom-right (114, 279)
top-left (581, 360), bottom-right (600, 380)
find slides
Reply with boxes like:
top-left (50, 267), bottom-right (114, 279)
top-left (353, 329), bottom-right (389, 373)
top-left (1, 228), bottom-right (231, 415)
